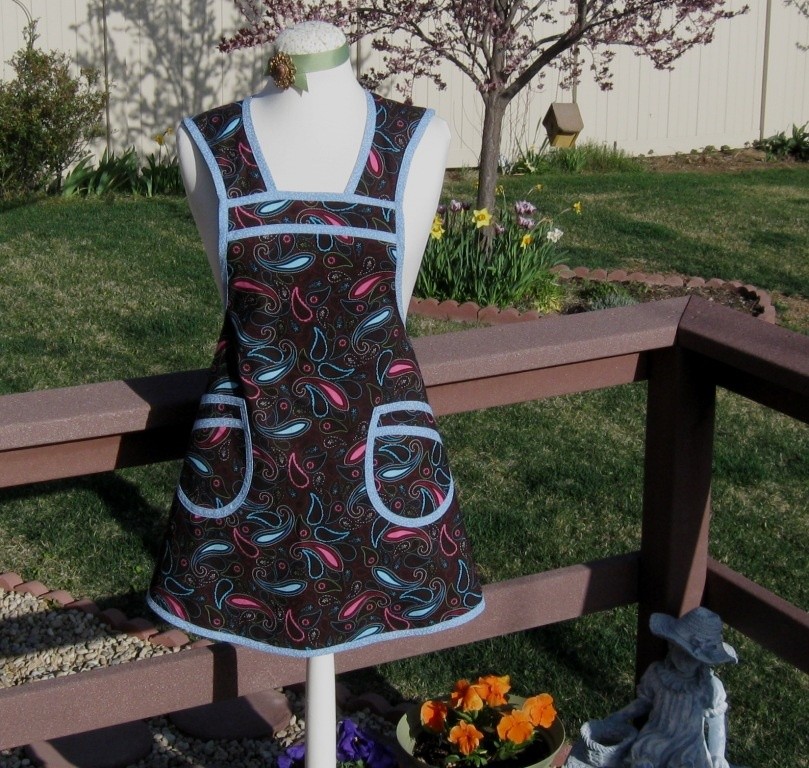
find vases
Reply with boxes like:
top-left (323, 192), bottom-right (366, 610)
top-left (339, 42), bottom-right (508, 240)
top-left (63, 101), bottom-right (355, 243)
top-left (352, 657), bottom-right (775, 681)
top-left (395, 696), bottom-right (565, 768)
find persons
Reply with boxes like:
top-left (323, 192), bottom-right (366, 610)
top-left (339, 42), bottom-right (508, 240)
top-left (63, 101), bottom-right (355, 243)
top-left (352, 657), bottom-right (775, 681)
top-left (147, 21), bottom-right (486, 658)
top-left (604, 607), bottom-right (738, 768)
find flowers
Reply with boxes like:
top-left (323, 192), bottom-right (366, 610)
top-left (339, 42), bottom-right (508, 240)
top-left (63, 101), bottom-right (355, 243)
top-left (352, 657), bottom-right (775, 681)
top-left (416, 674), bottom-right (557, 768)
